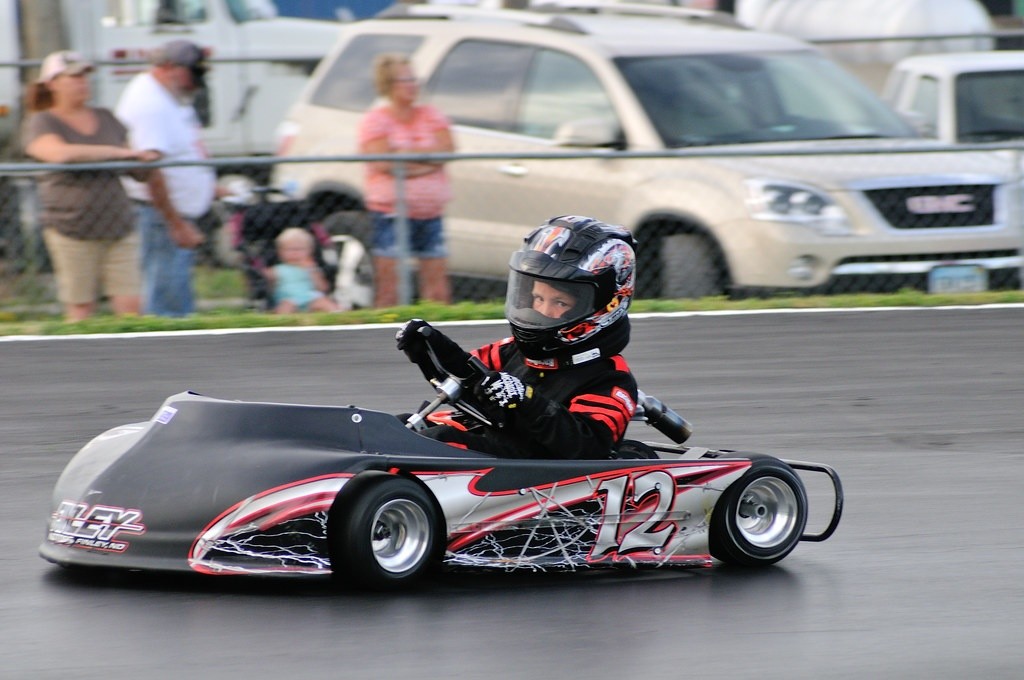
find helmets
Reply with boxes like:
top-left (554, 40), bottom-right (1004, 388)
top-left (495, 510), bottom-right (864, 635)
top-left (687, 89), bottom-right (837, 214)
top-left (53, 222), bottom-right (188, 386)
top-left (504, 215), bottom-right (638, 371)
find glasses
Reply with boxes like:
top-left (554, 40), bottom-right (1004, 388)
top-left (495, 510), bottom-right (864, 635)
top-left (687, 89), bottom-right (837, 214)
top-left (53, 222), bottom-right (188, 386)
top-left (391, 76), bottom-right (417, 81)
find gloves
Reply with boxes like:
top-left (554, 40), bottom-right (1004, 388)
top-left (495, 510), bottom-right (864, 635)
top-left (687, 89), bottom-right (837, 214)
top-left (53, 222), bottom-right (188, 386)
top-left (479, 371), bottom-right (525, 410)
top-left (395, 319), bottom-right (444, 364)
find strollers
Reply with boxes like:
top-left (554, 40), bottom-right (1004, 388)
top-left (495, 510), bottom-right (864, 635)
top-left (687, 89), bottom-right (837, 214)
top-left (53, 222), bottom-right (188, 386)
top-left (223, 187), bottom-right (337, 315)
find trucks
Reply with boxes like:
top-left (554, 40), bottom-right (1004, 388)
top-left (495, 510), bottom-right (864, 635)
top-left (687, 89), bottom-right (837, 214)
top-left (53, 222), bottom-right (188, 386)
top-left (1, 2), bottom-right (352, 272)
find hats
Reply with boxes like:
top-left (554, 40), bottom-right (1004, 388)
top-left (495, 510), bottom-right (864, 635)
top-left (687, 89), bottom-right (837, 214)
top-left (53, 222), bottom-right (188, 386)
top-left (149, 39), bottom-right (203, 68)
top-left (37, 49), bottom-right (96, 83)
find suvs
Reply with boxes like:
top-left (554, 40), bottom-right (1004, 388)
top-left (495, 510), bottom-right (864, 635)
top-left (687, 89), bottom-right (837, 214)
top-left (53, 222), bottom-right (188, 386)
top-left (266, 1), bottom-right (1024, 299)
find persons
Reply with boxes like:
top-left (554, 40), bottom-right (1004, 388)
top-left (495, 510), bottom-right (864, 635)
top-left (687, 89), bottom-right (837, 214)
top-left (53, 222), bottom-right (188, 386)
top-left (358, 51), bottom-right (456, 311)
top-left (260, 226), bottom-right (347, 316)
top-left (15, 49), bottom-right (166, 322)
top-left (110, 39), bottom-right (218, 319)
top-left (395, 214), bottom-right (638, 460)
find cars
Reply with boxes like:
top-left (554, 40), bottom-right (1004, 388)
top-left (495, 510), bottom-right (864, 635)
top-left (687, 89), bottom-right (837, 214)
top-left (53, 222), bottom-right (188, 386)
top-left (883, 51), bottom-right (1024, 202)
